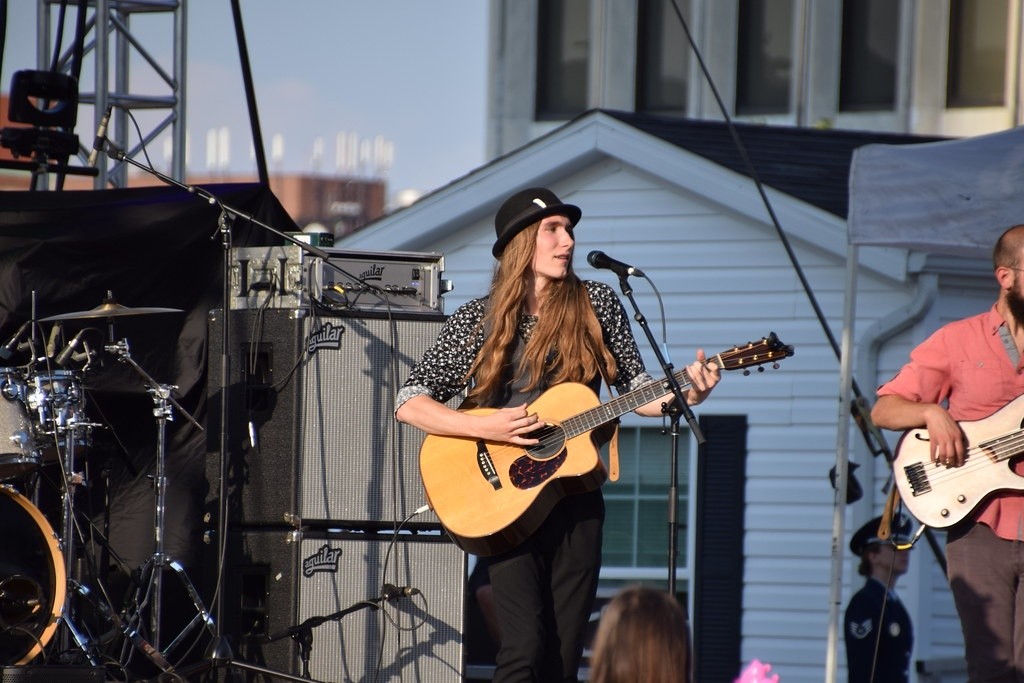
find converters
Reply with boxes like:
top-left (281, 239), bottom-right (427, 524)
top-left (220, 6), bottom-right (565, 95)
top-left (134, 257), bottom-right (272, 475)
top-left (284, 231), bottom-right (335, 248)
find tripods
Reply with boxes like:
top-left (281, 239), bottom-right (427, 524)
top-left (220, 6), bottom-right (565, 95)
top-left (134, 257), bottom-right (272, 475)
top-left (54, 317), bottom-right (323, 683)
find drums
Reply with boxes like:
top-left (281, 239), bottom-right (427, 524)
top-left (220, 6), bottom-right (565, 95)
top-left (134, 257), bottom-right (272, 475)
top-left (0, 367), bottom-right (42, 481)
top-left (22, 370), bottom-right (93, 467)
top-left (0, 484), bottom-right (67, 665)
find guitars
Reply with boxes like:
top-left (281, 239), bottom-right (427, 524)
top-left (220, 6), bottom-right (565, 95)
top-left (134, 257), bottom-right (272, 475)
top-left (892, 392), bottom-right (1024, 532)
top-left (418, 331), bottom-right (795, 557)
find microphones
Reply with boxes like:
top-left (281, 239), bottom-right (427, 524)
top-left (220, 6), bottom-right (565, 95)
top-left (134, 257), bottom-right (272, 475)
top-left (381, 583), bottom-right (420, 598)
top-left (0, 320), bottom-right (32, 360)
top-left (3, 598), bottom-right (39, 611)
top-left (55, 329), bottom-right (86, 366)
top-left (88, 105), bottom-right (112, 168)
top-left (586, 251), bottom-right (645, 277)
top-left (46, 322), bottom-right (60, 358)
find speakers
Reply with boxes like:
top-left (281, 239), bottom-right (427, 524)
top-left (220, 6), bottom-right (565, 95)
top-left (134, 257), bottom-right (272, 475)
top-left (201, 308), bottom-right (468, 683)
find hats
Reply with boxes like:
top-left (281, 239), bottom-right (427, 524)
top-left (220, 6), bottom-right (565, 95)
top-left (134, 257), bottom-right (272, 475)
top-left (492, 187), bottom-right (582, 260)
top-left (850, 513), bottom-right (912, 557)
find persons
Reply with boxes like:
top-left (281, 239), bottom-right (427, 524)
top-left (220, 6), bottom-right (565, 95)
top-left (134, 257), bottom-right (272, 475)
top-left (871, 224), bottom-right (1024, 683)
top-left (589, 585), bottom-right (692, 683)
top-left (844, 513), bottom-right (914, 683)
top-left (392, 186), bottom-right (722, 683)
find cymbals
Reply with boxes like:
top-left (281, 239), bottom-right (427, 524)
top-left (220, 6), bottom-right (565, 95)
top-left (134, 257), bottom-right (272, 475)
top-left (38, 303), bottom-right (185, 321)
